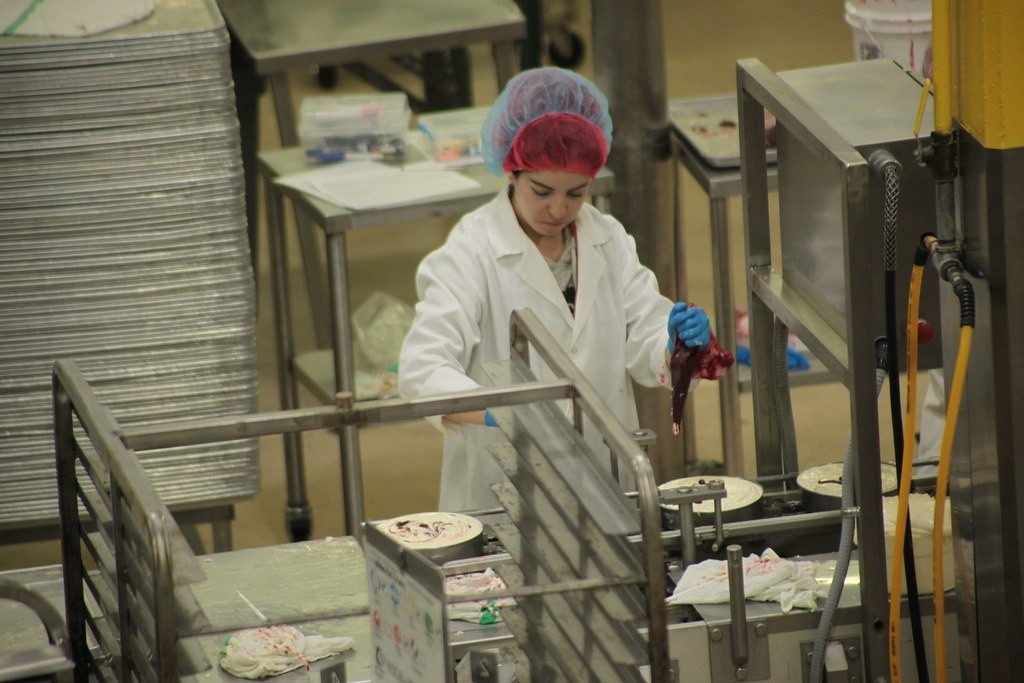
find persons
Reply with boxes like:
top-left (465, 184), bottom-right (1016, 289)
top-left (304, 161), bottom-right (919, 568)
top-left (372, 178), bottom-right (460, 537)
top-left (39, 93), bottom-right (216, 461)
top-left (396, 67), bottom-right (712, 514)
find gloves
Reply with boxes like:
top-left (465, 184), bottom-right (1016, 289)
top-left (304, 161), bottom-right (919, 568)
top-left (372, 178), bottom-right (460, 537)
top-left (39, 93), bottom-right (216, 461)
top-left (735, 346), bottom-right (810, 370)
top-left (483, 410), bottom-right (497, 426)
top-left (667, 301), bottom-right (710, 354)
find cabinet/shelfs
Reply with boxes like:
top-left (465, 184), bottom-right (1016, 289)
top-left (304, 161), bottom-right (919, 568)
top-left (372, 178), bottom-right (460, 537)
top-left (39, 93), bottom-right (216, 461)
top-left (258, 107), bottom-right (615, 538)
top-left (673, 97), bottom-right (828, 478)
